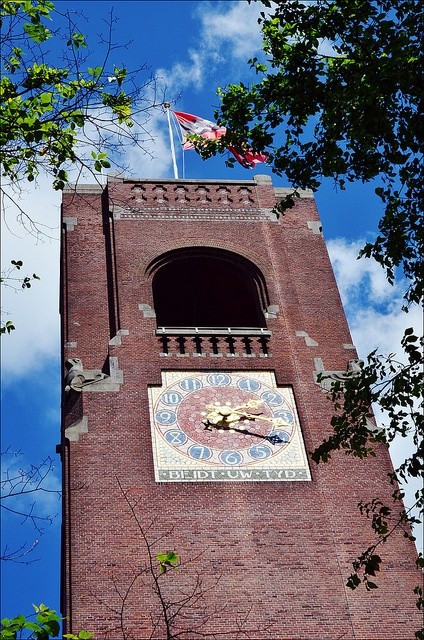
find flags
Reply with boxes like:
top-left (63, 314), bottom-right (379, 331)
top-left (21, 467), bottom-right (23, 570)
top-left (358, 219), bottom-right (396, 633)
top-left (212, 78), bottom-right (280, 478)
top-left (170, 108), bottom-right (269, 169)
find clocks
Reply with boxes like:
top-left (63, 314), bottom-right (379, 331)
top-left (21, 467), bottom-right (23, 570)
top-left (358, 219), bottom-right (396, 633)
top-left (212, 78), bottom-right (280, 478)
top-left (144, 369), bottom-right (313, 482)
